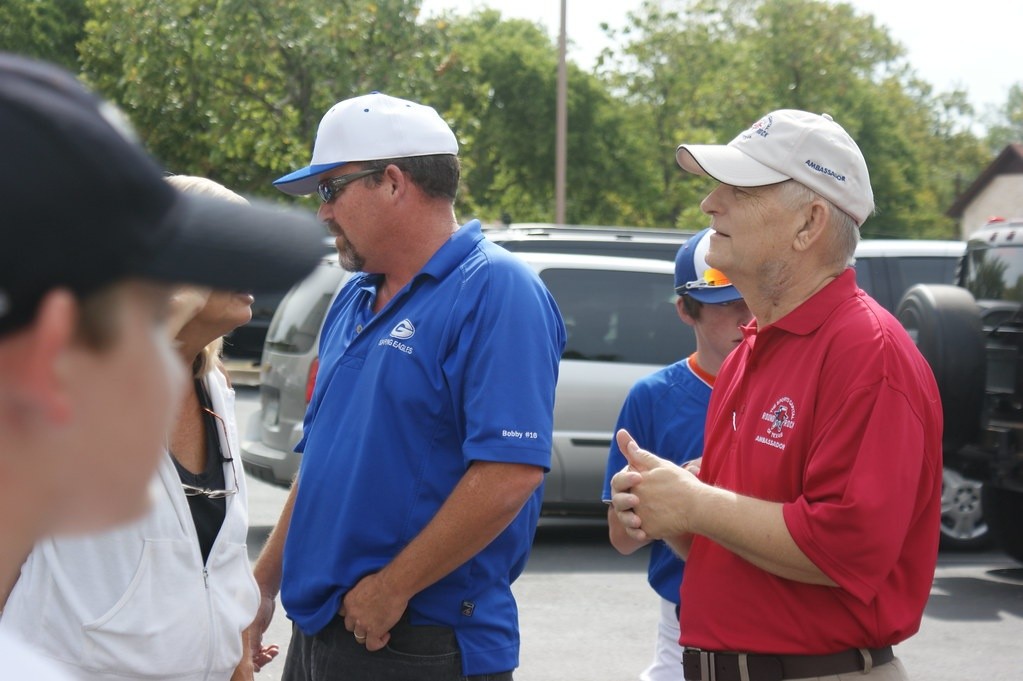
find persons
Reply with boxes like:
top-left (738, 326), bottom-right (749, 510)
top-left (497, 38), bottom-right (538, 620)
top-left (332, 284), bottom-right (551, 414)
top-left (602, 226), bottom-right (755, 681)
top-left (0, 174), bottom-right (262, 681)
top-left (0, 51), bottom-right (327, 681)
top-left (610, 108), bottom-right (944, 681)
top-left (232, 90), bottom-right (567, 680)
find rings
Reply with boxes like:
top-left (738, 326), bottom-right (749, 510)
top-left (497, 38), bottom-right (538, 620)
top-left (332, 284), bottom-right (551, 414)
top-left (353, 630), bottom-right (365, 639)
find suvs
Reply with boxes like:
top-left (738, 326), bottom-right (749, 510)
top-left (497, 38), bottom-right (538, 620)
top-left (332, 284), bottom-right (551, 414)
top-left (854, 217), bottom-right (1023, 561)
top-left (239, 222), bottom-right (702, 528)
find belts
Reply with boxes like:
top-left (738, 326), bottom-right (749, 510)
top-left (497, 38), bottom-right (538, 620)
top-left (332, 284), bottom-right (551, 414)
top-left (683, 645), bottom-right (894, 681)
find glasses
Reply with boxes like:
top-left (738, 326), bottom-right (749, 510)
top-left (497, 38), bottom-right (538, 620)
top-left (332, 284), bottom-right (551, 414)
top-left (316, 168), bottom-right (385, 204)
top-left (675, 266), bottom-right (732, 293)
top-left (180, 407), bottom-right (239, 501)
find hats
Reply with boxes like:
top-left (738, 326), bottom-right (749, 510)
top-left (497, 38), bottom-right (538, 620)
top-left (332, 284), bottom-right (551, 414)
top-left (675, 109), bottom-right (874, 227)
top-left (1, 49), bottom-right (330, 340)
top-left (674, 227), bottom-right (744, 305)
top-left (273, 90), bottom-right (460, 196)
